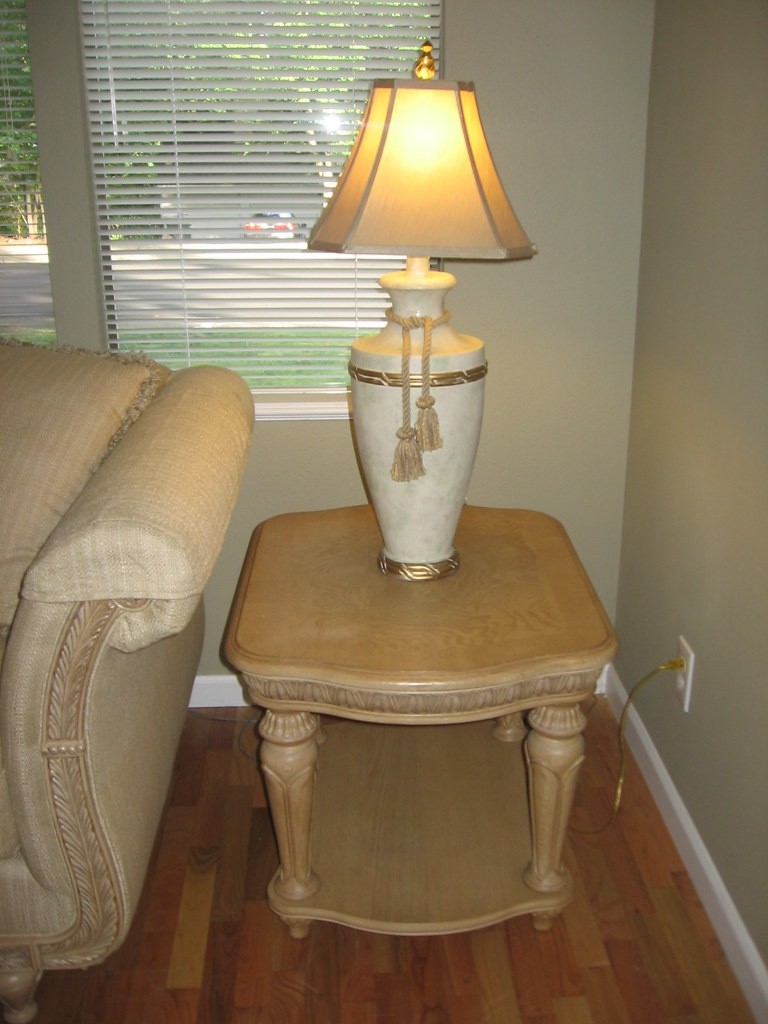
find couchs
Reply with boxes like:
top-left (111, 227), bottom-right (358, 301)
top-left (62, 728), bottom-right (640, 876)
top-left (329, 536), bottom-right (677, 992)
top-left (2, 367), bottom-right (256, 1023)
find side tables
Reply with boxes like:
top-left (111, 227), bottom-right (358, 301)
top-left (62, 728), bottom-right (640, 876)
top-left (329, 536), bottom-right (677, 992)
top-left (222, 505), bottom-right (618, 938)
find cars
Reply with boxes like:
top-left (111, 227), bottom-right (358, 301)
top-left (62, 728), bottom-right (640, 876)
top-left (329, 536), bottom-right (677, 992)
top-left (243, 210), bottom-right (307, 239)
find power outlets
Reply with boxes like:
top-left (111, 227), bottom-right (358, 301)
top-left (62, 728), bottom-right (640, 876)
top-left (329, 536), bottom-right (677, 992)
top-left (672, 635), bottom-right (695, 713)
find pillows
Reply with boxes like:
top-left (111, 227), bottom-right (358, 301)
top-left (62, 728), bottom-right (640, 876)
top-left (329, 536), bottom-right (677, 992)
top-left (0, 336), bottom-right (171, 639)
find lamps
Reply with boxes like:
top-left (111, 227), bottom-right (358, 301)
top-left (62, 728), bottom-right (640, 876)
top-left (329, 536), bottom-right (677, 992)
top-left (308, 39), bottom-right (541, 581)
